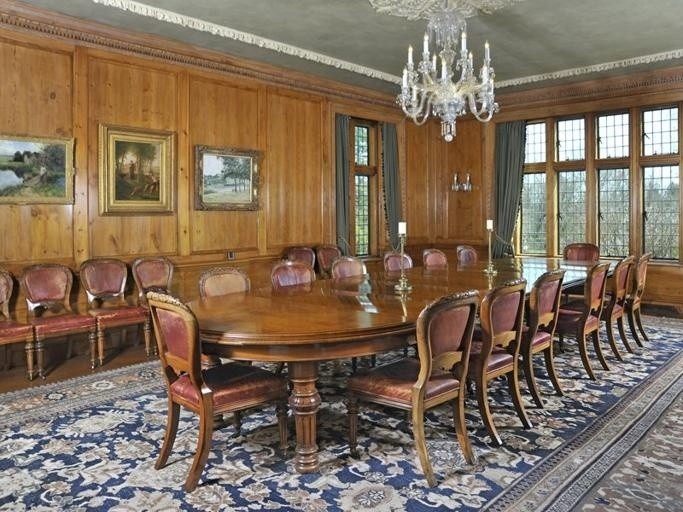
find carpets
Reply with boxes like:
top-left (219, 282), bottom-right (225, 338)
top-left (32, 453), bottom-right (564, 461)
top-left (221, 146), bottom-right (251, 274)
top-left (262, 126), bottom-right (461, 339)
top-left (0, 317), bottom-right (683, 509)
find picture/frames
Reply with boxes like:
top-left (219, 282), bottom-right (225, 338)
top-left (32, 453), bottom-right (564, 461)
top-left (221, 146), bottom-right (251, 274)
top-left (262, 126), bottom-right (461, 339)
top-left (97, 122), bottom-right (178, 216)
top-left (193, 144), bottom-right (264, 211)
top-left (0, 134), bottom-right (76, 206)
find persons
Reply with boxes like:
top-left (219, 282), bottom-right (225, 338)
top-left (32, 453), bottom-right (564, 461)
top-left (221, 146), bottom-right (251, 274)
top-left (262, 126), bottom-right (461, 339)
top-left (128, 159), bottom-right (158, 196)
top-left (34, 164), bottom-right (47, 185)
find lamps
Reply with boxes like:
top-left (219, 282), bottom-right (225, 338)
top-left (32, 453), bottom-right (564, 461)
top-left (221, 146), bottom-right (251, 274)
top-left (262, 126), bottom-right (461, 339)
top-left (450, 172), bottom-right (475, 193)
top-left (368, 0), bottom-right (511, 145)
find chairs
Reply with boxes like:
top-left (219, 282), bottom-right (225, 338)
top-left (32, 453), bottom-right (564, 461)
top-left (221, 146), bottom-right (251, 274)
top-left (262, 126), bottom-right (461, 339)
top-left (280, 244), bottom-right (316, 270)
top-left (382, 250), bottom-right (414, 271)
top-left (0, 264), bottom-right (36, 382)
top-left (80, 257), bottom-right (151, 367)
top-left (198, 265), bottom-right (254, 296)
top-left (456, 244), bottom-right (479, 265)
top-left (329, 254), bottom-right (368, 280)
top-left (496, 268), bottom-right (569, 410)
top-left (342, 288), bottom-right (483, 490)
top-left (592, 253), bottom-right (635, 363)
top-left (421, 247), bottom-right (448, 266)
top-left (272, 260), bottom-right (316, 288)
top-left (622, 252), bottom-right (654, 349)
top-left (144, 290), bottom-right (292, 493)
top-left (21, 261), bottom-right (98, 379)
top-left (315, 244), bottom-right (344, 278)
top-left (452, 278), bottom-right (533, 449)
top-left (132, 252), bottom-right (175, 357)
top-left (553, 262), bottom-right (613, 383)
top-left (563, 241), bottom-right (601, 263)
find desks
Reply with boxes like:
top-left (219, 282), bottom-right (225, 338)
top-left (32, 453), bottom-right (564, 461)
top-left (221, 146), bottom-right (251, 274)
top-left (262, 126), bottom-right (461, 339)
top-left (184, 254), bottom-right (638, 474)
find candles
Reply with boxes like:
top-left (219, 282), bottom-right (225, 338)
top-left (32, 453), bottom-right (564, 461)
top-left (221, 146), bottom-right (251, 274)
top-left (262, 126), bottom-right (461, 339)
top-left (397, 222), bottom-right (407, 234)
top-left (486, 219), bottom-right (493, 229)
